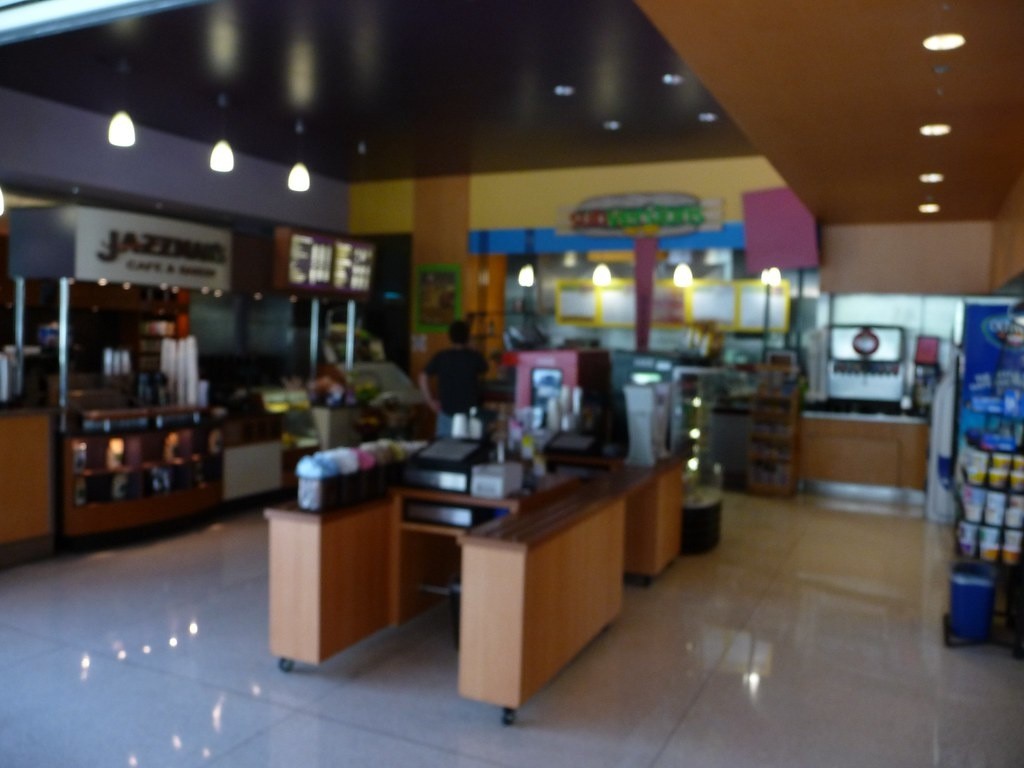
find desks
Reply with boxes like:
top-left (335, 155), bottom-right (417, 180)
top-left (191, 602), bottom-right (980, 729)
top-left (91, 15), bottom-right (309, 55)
top-left (801, 411), bottom-right (927, 507)
top-left (262, 451), bottom-right (685, 726)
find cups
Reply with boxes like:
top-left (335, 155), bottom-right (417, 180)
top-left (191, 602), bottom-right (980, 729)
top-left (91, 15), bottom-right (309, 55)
top-left (547, 383), bottom-right (584, 433)
top-left (0, 345), bottom-right (16, 403)
top-left (957, 451), bottom-right (1024, 565)
top-left (103, 347), bottom-right (130, 377)
top-left (451, 413), bottom-right (483, 439)
top-left (160, 334), bottom-right (208, 407)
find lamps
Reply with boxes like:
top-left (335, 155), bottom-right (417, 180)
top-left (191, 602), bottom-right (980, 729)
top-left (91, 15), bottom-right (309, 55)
top-left (107, 92), bottom-right (310, 192)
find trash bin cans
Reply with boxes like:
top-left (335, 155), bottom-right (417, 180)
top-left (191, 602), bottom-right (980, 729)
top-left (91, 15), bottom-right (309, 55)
top-left (681, 487), bottom-right (722, 554)
top-left (952, 562), bottom-right (995, 639)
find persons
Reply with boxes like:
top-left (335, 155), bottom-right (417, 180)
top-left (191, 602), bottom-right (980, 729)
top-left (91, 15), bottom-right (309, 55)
top-left (420, 320), bottom-right (490, 439)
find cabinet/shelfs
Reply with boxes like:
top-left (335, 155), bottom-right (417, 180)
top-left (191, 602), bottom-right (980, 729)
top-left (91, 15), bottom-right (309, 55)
top-left (741, 362), bottom-right (808, 496)
top-left (62, 401), bottom-right (222, 553)
